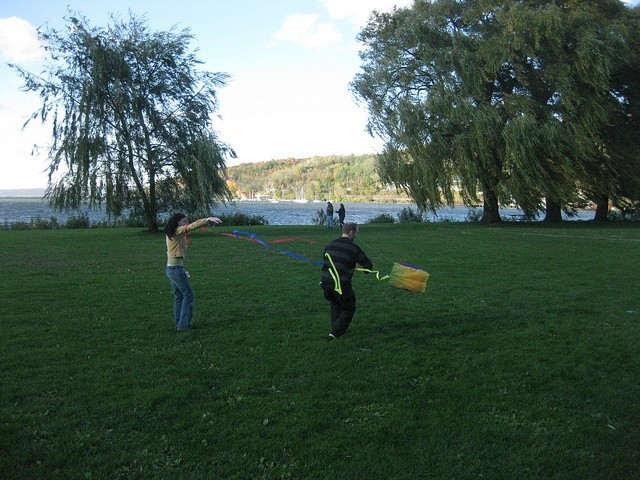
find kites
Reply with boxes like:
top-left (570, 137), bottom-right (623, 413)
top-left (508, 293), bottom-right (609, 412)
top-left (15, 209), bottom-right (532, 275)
top-left (184, 223), bottom-right (430, 294)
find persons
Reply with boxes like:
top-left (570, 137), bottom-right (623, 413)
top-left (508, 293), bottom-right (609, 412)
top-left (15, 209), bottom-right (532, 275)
top-left (326, 201), bottom-right (333, 230)
top-left (336, 204), bottom-right (345, 231)
top-left (166, 214), bottom-right (222, 331)
top-left (320, 222), bottom-right (372, 340)
